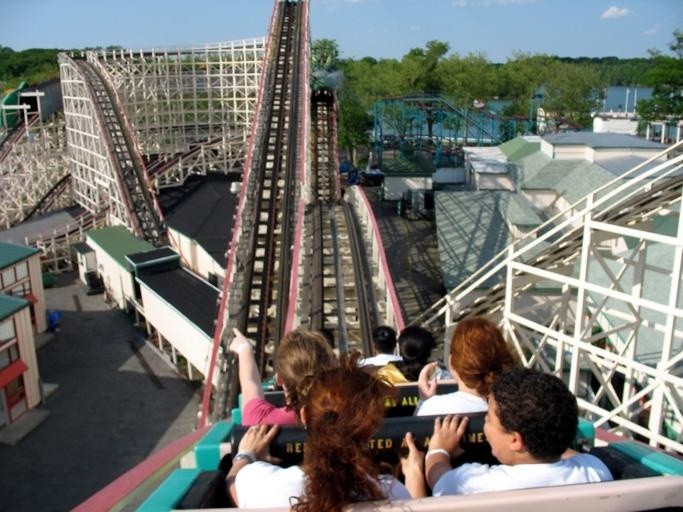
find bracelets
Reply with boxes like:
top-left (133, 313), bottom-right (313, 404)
top-left (417, 399), bottom-right (424, 409)
top-left (425, 448), bottom-right (450, 462)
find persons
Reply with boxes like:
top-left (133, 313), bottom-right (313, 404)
top-left (424, 367), bottom-right (614, 496)
top-left (356, 326), bottom-right (404, 369)
top-left (370, 326), bottom-right (436, 387)
top-left (230, 327), bottom-right (341, 426)
top-left (316, 329), bottom-right (336, 348)
top-left (224, 349), bottom-right (426, 512)
top-left (413, 318), bottom-right (521, 416)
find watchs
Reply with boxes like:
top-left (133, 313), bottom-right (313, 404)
top-left (232, 450), bottom-right (256, 463)
